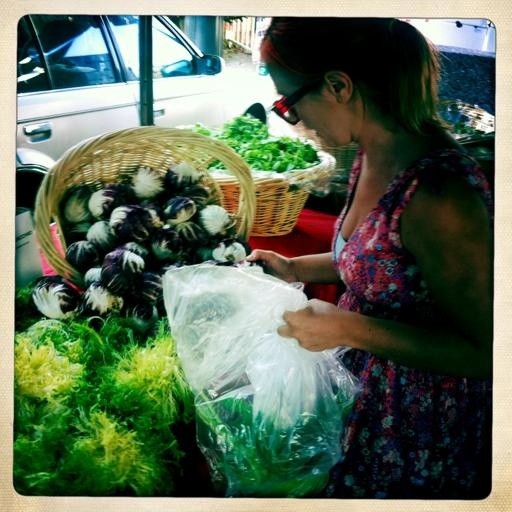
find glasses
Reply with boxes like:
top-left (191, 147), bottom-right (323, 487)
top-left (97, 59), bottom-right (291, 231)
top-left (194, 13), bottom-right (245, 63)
top-left (267, 78), bottom-right (325, 125)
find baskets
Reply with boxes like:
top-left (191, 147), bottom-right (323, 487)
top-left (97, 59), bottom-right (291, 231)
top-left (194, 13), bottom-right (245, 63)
top-left (210, 148), bottom-right (337, 236)
top-left (292, 122), bottom-right (359, 171)
top-left (34, 126), bottom-right (257, 290)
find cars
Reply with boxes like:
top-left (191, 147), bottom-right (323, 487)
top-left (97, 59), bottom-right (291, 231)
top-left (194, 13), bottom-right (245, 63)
top-left (18, 16), bottom-right (272, 211)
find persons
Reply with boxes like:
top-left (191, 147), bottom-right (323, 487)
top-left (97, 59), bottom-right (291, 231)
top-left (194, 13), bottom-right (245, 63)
top-left (236, 17), bottom-right (491, 499)
